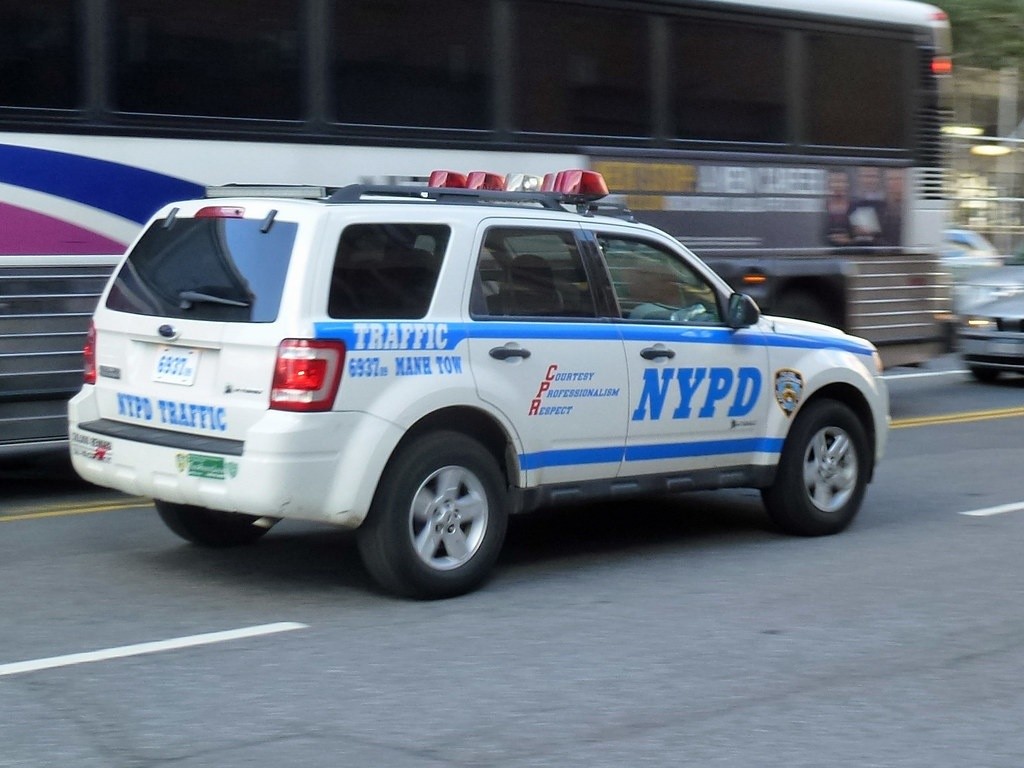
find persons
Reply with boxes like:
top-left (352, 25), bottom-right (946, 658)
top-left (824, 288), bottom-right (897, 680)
top-left (827, 166), bottom-right (903, 247)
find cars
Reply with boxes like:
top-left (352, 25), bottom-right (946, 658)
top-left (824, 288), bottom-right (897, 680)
top-left (951, 241), bottom-right (1024, 381)
top-left (66, 168), bottom-right (892, 602)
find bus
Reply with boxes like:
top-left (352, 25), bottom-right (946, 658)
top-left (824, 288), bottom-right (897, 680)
top-left (0, 2), bottom-right (958, 470)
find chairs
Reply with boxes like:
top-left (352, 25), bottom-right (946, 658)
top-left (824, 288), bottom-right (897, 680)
top-left (359, 249), bottom-right (428, 312)
top-left (504, 254), bottom-right (563, 315)
